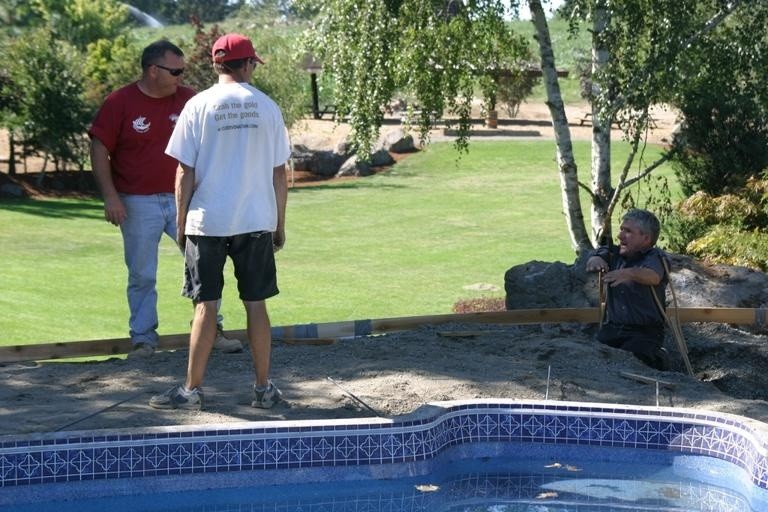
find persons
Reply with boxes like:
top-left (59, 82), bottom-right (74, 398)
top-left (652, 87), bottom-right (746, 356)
top-left (583, 208), bottom-right (673, 372)
top-left (87, 40), bottom-right (244, 356)
top-left (149, 32), bottom-right (293, 411)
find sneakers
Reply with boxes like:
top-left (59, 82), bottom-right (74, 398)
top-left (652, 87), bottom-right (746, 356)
top-left (249, 379), bottom-right (283, 410)
top-left (212, 321), bottom-right (245, 352)
top-left (125, 340), bottom-right (155, 358)
top-left (148, 383), bottom-right (203, 411)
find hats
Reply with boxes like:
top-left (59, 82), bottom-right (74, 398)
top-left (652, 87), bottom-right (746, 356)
top-left (211, 33), bottom-right (265, 67)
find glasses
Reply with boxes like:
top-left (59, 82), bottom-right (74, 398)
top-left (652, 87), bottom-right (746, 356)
top-left (147, 63), bottom-right (188, 77)
